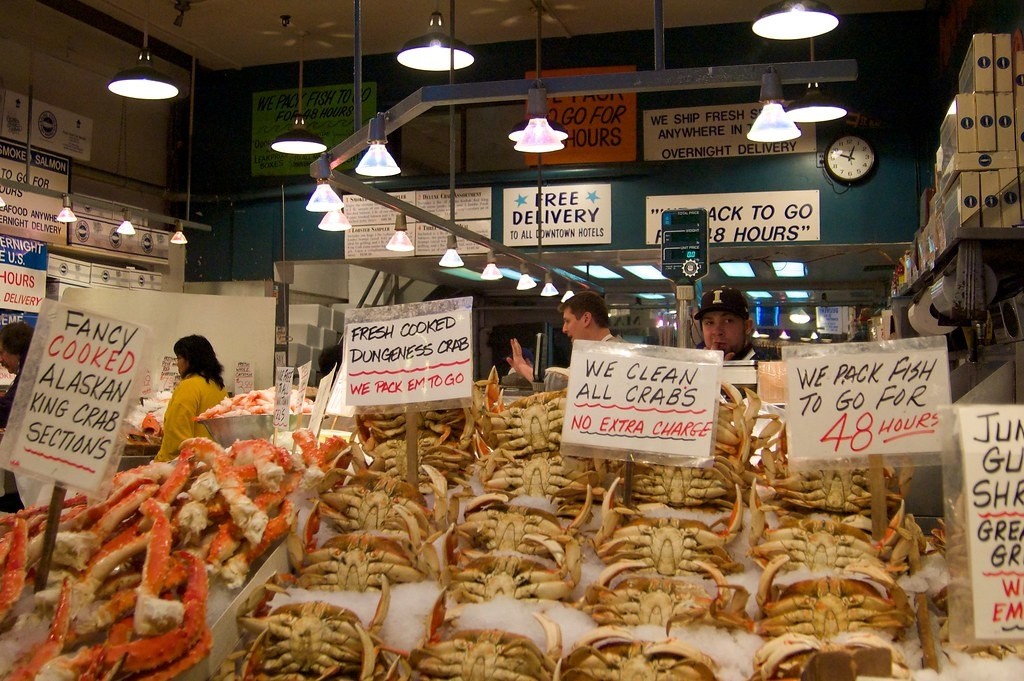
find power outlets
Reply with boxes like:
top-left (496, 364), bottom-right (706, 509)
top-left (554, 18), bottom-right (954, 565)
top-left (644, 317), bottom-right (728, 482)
top-left (816, 152), bottom-right (825, 168)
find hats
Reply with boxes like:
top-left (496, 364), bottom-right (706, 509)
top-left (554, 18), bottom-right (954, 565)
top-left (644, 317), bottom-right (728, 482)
top-left (695, 287), bottom-right (750, 320)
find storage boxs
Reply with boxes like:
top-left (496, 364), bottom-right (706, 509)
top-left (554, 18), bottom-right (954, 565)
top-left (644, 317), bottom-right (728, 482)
top-left (0, 88), bottom-right (169, 325)
top-left (916, 31), bottom-right (1024, 268)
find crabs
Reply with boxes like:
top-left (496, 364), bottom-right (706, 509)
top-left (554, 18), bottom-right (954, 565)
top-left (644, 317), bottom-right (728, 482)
top-left (0, 412), bottom-right (349, 681)
top-left (194, 384), bottom-right (317, 419)
top-left (220, 362), bottom-right (1024, 681)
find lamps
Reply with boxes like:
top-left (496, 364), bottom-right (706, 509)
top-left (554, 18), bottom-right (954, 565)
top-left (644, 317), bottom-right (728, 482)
top-left (58, 196), bottom-right (77, 222)
top-left (271, 0), bottom-right (846, 331)
top-left (173, 0), bottom-right (193, 28)
top-left (108, 14), bottom-right (179, 100)
top-left (117, 208), bottom-right (136, 234)
top-left (170, 220), bottom-right (188, 244)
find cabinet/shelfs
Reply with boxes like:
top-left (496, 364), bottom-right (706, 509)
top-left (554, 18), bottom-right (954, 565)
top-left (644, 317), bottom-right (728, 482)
top-left (895, 227), bottom-right (1024, 404)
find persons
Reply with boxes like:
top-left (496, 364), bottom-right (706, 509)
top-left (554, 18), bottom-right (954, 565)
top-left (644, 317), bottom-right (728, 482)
top-left (153, 334), bottom-right (230, 464)
top-left (506, 291), bottom-right (628, 385)
top-left (0, 321), bottom-right (35, 513)
top-left (694, 284), bottom-right (782, 361)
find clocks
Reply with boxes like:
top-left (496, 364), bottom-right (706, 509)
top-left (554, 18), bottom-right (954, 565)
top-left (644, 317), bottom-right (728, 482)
top-left (825, 134), bottom-right (876, 183)
